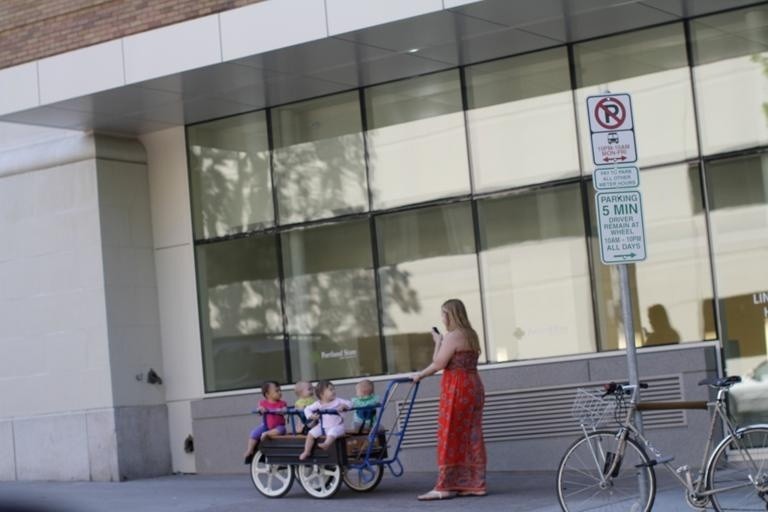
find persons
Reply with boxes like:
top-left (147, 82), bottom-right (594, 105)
top-left (293, 379), bottom-right (320, 433)
top-left (298, 381), bottom-right (354, 460)
top-left (406, 298), bottom-right (488, 501)
top-left (350, 379), bottom-right (384, 432)
top-left (244, 380), bottom-right (287, 460)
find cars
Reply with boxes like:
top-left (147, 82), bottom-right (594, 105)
top-left (729, 358), bottom-right (768, 417)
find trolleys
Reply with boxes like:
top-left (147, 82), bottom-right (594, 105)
top-left (244, 377), bottom-right (421, 499)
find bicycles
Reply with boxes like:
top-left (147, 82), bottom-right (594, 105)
top-left (556, 376), bottom-right (768, 512)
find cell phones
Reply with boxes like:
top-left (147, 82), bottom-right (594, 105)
top-left (432, 327), bottom-right (440, 335)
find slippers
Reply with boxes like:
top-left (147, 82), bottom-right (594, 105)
top-left (416, 487), bottom-right (487, 501)
